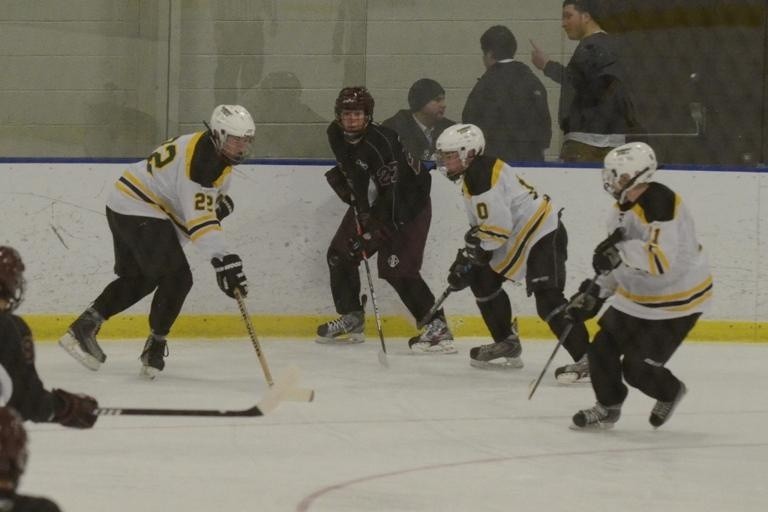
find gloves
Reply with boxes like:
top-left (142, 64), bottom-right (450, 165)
top-left (215, 194), bottom-right (234, 221)
top-left (51, 388), bottom-right (98, 428)
top-left (447, 247), bottom-right (493, 289)
top-left (563, 279), bottom-right (608, 325)
top-left (344, 231), bottom-right (378, 260)
top-left (210, 254), bottom-right (248, 299)
top-left (592, 233), bottom-right (622, 275)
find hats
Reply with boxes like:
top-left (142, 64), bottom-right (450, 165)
top-left (408, 78), bottom-right (443, 111)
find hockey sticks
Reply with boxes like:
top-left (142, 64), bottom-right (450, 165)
top-left (411, 283), bottom-right (456, 357)
top-left (91, 370), bottom-right (308, 419)
top-left (226, 274), bottom-right (318, 406)
top-left (524, 269), bottom-right (601, 402)
top-left (343, 183), bottom-right (407, 372)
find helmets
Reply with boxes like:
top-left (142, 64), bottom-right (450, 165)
top-left (334, 87), bottom-right (375, 144)
top-left (601, 141), bottom-right (658, 204)
top-left (209, 104), bottom-right (256, 165)
top-left (0, 407), bottom-right (28, 477)
top-left (0, 245), bottom-right (25, 312)
top-left (435, 123), bottom-right (486, 181)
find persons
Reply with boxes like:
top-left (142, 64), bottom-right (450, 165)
top-left (462, 26), bottom-right (551, 168)
top-left (433, 124), bottom-right (592, 381)
top-left (530, 2), bottom-right (642, 164)
top-left (66, 104), bottom-right (257, 373)
top-left (382, 78), bottom-right (460, 167)
top-left (566, 142), bottom-right (715, 428)
top-left (1, 246), bottom-right (100, 511)
top-left (316, 87), bottom-right (459, 347)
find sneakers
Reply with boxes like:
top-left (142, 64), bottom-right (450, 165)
top-left (409, 307), bottom-right (454, 348)
top-left (69, 306), bottom-right (107, 363)
top-left (318, 310), bottom-right (365, 338)
top-left (650, 400), bottom-right (673, 427)
top-left (555, 353), bottom-right (592, 383)
top-left (470, 316), bottom-right (522, 361)
top-left (140, 334), bottom-right (168, 370)
top-left (573, 401), bottom-right (622, 428)
